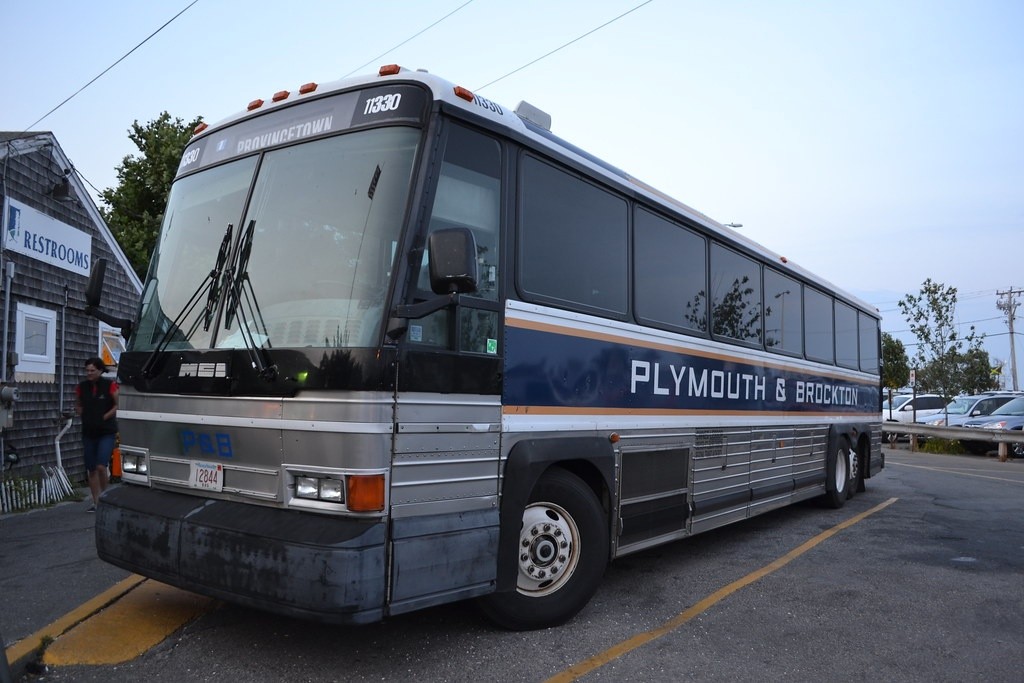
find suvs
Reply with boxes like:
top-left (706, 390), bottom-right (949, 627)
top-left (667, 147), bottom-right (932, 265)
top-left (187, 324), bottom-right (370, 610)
top-left (907, 390), bottom-right (1024, 443)
top-left (881, 393), bottom-right (959, 444)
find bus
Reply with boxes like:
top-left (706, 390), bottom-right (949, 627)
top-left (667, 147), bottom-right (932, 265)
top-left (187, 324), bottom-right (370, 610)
top-left (82, 60), bottom-right (886, 638)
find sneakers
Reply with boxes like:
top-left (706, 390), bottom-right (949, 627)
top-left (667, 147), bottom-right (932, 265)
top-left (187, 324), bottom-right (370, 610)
top-left (85, 506), bottom-right (97, 512)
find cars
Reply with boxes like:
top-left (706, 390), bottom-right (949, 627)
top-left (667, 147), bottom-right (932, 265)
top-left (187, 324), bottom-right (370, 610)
top-left (960, 396), bottom-right (1024, 458)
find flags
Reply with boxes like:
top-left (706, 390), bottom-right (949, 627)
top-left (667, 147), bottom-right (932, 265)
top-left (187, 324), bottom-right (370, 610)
top-left (990, 366), bottom-right (1002, 375)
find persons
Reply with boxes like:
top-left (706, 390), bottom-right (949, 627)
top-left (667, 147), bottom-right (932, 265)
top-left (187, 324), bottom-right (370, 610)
top-left (72, 357), bottom-right (119, 513)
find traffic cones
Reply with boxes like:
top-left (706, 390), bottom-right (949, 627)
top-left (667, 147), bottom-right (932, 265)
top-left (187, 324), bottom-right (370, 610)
top-left (107, 448), bottom-right (123, 483)
